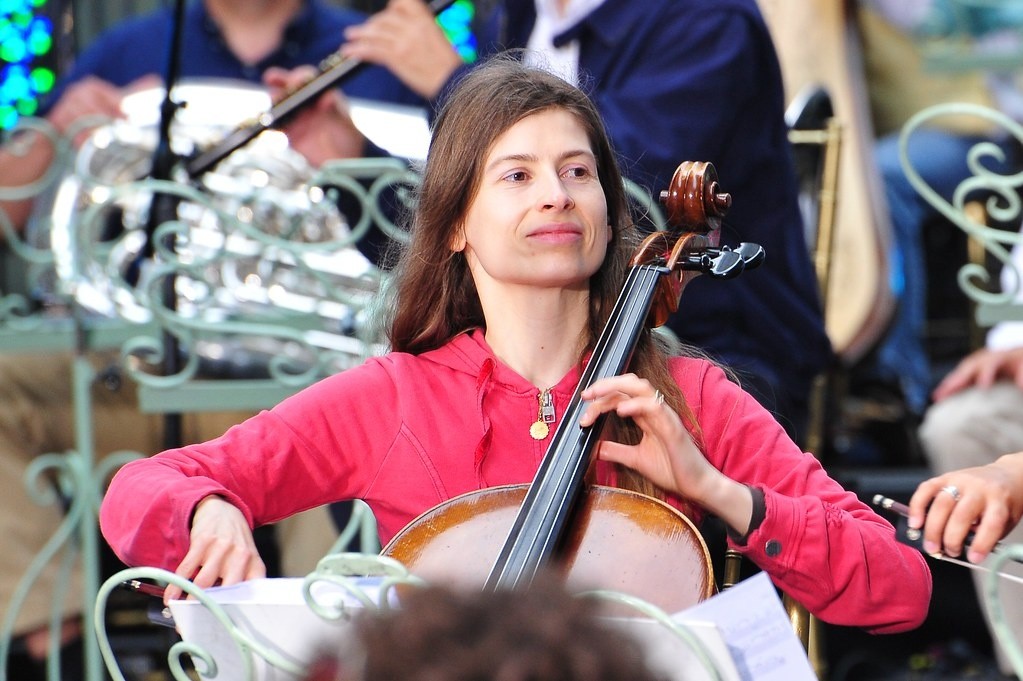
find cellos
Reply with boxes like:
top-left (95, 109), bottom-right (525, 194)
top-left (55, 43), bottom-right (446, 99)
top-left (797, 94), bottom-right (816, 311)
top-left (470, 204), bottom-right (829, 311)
top-left (376, 162), bottom-right (776, 624)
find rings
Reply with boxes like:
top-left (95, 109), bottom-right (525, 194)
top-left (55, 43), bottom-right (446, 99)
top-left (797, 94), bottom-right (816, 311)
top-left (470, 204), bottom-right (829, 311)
top-left (655, 389), bottom-right (664, 404)
top-left (942, 486), bottom-right (958, 498)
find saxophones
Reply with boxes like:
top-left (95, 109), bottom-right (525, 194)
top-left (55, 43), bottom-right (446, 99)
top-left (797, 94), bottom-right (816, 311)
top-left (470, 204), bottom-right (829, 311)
top-left (53, 78), bottom-right (435, 361)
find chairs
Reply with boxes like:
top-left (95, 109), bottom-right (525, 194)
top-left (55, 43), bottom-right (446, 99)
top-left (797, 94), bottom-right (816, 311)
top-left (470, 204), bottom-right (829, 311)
top-left (720, 114), bottom-right (844, 681)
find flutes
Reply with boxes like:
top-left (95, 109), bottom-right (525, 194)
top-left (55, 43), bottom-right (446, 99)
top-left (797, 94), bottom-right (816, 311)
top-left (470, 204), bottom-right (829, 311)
top-left (187, 0), bottom-right (456, 179)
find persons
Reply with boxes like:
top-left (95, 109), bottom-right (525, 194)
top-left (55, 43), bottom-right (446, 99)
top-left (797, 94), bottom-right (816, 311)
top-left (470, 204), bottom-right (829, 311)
top-left (98, 59), bottom-right (932, 633)
top-left (263, 0), bottom-right (839, 431)
top-left (908, 344), bottom-right (1023, 681)
top-left (0, 0), bottom-right (433, 269)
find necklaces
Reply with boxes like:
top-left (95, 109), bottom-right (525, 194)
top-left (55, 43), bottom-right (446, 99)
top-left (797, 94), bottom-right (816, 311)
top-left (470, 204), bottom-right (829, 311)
top-left (530, 388), bottom-right (548, 439)
